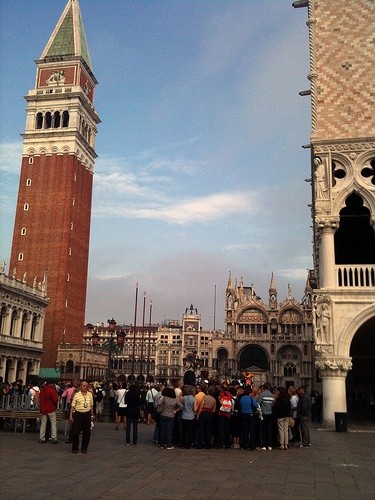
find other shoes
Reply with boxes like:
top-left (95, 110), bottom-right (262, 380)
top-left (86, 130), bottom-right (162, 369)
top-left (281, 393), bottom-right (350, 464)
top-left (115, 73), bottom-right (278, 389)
top-left (82, 450), bottom-right (87, 453)
top-left (161, 446), bottom-right (174, 450)
top-left (127, 443), bottom-right (130, 446)
top-left (65, 440), bottom-right (73, 443)
top-left (39, 439), bottom-right (47, 443)
top-left (51, 438), bottom-right (59, 443)
top-left (133, 442), bottom-right (137, 446)
top-left (115, 427), bottom-right (118, 430)
top-left (47, 438), bottom-right (51, 441)
top-left (73, 450), bottom-right (77, 454)
top-left (95, 416), bottom-right (155, 425)
top-left (177, 442), bottom-right (312, 451)
top-left (152, 440), bottom-right (159, 443)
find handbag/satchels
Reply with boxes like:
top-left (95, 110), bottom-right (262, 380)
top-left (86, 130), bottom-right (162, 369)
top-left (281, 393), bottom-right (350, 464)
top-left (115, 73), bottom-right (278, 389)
top-left (151, 407), bottom-right (161, 421)
top-left (289, 430), bottom-right (293, 440)
top-left (112, 402), bottom-right (120, 413)
top-left (153, 397), bottom-right (158, 408)
top-left (251, 397), bottom-right (257, 416)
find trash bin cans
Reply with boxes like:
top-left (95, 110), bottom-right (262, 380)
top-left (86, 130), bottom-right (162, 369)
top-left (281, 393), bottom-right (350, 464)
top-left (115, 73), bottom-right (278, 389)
top-left (334, 412), bottom-right (348, 432)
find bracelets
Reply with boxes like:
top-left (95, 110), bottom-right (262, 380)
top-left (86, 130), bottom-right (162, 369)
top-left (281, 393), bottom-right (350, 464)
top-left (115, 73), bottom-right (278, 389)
top-left (91, 415), bottom-right (94, 418)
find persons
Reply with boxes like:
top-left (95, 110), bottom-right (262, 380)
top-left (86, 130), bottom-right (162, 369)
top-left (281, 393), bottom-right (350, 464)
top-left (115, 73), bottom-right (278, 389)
top-left (68, 380), bottom-right (94, 454)
top-left (38, 380), bottom-right (59, 445)
top-left (124, 384), bottom-right (140, 448)
top-left (0, 376), bottom-right (312, 450)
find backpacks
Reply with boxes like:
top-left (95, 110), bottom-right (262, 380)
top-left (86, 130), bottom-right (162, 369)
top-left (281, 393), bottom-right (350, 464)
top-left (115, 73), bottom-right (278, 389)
top-left (96, 389), bottom-right (105, 404)
top-left (29, 388), bottom-right (40, 407)
top-left (231, 397), bottom-right (240, 417)
